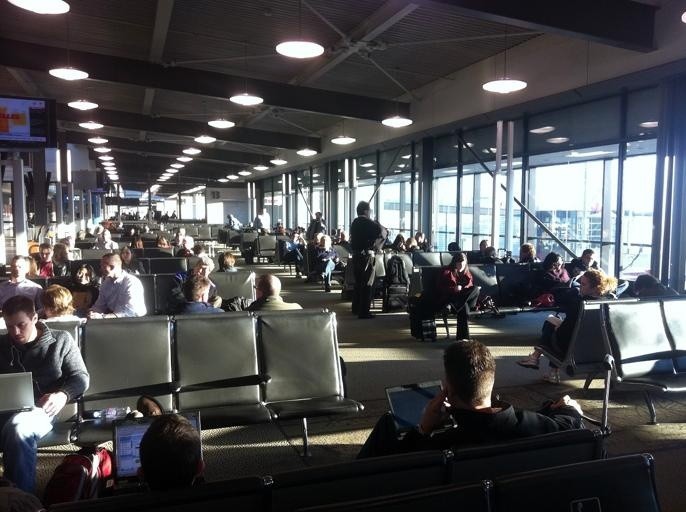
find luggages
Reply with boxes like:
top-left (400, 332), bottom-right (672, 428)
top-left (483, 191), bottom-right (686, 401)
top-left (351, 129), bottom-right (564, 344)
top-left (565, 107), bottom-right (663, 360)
top-left (410, 299), bottom-right (437, 340)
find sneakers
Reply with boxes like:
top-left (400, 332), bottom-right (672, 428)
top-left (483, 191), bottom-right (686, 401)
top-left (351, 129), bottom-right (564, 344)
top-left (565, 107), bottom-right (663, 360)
top-left (316, 274), bottom-right (331, 292)
top-left (515, 354), bottom-right (539, 370)
top-left (543, 372), bottom-right (560, 385)
top-left (136, 395), bottom-right (164, 416)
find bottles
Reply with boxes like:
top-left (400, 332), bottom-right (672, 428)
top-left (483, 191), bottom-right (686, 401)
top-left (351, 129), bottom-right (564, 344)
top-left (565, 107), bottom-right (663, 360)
top-left (91, 408), bottom-right (131, 419)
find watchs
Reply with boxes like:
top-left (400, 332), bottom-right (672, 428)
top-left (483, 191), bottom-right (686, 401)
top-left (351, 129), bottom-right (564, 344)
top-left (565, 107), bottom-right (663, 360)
top-left (414, 422), bottom-right (430, 437)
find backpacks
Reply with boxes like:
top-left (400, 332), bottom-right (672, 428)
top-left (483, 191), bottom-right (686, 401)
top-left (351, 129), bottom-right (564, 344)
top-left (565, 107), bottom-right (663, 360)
top-left (41, 446), bottom-right (117, 504)
top-left (383, 256), bottom-right (410, 293)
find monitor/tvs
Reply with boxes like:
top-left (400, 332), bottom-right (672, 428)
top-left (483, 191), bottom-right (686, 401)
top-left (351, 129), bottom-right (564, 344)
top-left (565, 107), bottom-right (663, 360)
top-left (0, 95), bottom-right (56, 148)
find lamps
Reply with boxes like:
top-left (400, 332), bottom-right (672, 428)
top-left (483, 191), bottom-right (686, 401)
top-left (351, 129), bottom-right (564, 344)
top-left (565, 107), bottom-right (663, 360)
top-left (78, 121), bottom-right (105, 130)
top-left (482, 27), bottom-right (528, 95)
top-left (381, 87), bottom-right (414, 128)
top-left (329, 119), bottom-right (357, 146)
top-left (87, 135), bottom-right (109, 144)
top-left (207, 102), bottom-right (236, 129)
top-left (7, 0), bottom-right (71, 15)
top-left (194, 134), bottom-right (217, 144)
top-left (48, 13), bottom-right (90, 81)
top-left (276, 1), bottom-right (326, 59)
top-left (230, 45), bottom-right (264, 106)
top-left (67, 98), bottom-right (99, 111)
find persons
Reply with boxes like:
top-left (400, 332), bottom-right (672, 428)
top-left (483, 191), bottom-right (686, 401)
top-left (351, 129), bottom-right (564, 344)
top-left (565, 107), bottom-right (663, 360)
top-left (0, 295), bottom-right (90, 494)
top-left (44, 394), bottom-right (206, 509)
top-left (357, 339), bottom-right (584, 461)
top-left (0, 475), bottom-right (17, 488)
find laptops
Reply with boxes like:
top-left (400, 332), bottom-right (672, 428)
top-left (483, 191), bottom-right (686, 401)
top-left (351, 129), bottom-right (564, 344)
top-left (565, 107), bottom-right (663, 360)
top-left (112, 410), bottom-right (204, 496)
top-left (0, 372), bottom-right (34, 412)
top-left (386, 380), bottom-right (459, 441)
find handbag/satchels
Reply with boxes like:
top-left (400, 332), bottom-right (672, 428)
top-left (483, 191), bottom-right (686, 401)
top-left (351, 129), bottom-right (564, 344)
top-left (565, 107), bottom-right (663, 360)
top-left (477, 295), bottom-right (497, 312)
top-left (531, 293), bottom-right (556, 308)
top-left (384, 283), bottom-right (408, 312)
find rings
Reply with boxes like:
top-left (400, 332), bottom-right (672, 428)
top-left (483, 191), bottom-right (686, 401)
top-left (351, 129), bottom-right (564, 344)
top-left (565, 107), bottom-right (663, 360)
top-left (50, 410), bottom-right (54, 414)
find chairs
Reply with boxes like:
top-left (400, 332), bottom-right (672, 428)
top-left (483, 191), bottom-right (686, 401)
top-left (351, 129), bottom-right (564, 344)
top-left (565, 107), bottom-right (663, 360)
top-left (533, 295), bottom-right (686, 431)
top-left (47, 428), bottom-right (660, 511)
top-left (371, 248), bottom-right (572, 340)
top-left (0, 220), bottom-right (365, 460)
